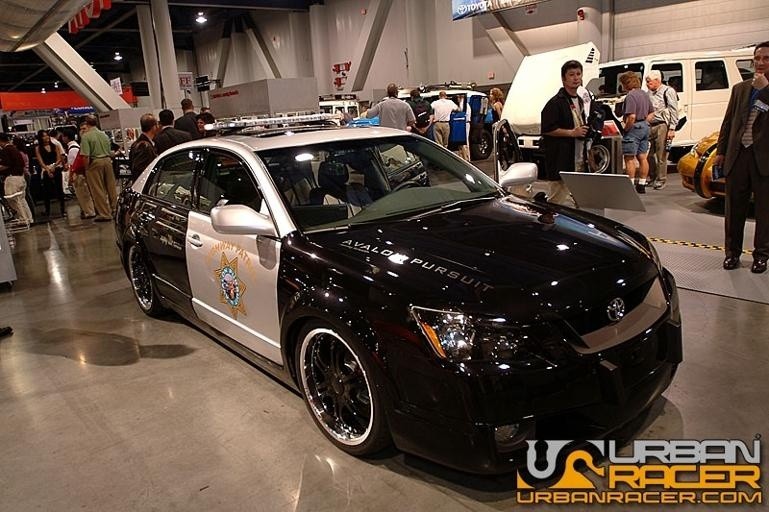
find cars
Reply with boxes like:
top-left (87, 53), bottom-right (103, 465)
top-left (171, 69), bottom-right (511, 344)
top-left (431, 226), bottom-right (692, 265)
top-left (111, 124), bottom-right (684, 476)
top-left (676, 129), bottom-right (754, 208)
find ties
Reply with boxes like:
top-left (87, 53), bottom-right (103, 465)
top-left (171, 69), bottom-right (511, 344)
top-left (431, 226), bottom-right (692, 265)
top-left (741, 107), bottom-right (761, 148)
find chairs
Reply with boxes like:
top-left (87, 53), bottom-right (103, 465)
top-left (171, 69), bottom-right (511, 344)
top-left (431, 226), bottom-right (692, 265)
top-left (312, 160), bottom-right (375, 219)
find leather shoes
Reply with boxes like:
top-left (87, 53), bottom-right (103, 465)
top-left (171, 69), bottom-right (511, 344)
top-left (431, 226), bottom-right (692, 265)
top-left (751, 259), bottom-right (767, 273)
top-left (723, 255), bottom-right (739, 269)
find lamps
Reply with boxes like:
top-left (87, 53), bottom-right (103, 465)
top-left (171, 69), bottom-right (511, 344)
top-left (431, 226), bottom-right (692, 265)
top-left (111, 47), bottom-right (124, 62)
top-left (194, 7), bottom-right (210, 24)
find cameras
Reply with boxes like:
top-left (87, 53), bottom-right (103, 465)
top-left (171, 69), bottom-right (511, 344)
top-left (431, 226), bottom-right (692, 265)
top-left (578, 125), bottom-right (594, 141)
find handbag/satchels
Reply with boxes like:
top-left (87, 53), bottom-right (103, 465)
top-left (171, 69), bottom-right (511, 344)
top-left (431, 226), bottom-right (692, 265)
top-left (662, 100), bottom-right (686, 131)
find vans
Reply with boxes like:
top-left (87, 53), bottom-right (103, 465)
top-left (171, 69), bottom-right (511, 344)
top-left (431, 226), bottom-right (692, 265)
top-left (492, 46), bottom-right (758, 174)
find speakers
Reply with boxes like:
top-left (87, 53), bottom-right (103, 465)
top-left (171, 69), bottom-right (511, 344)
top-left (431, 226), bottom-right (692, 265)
top-left (131, 82), bottom-right (150, 96)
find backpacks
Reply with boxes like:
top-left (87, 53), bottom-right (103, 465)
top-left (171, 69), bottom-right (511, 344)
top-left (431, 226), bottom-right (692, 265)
top-left (413, 99), bottom-right (430, 127)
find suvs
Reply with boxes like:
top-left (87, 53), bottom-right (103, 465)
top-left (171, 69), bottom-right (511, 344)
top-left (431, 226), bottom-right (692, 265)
top-left (54, 125), bottom-right (79, 143)
top-left (378, 79), bottom-right (494, 159)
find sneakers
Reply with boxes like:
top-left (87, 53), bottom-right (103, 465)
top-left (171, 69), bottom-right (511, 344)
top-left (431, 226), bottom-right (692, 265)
top-left (654, 182), bottom-right (666, 190)
top-left (636, 182), bottom-right (647, 194)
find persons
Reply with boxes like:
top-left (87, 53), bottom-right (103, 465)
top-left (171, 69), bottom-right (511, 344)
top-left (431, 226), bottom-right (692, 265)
top-left (0, 69), bottom-right (693, 226)
top-left (712, 41), bottom-right (769, 273)
top-left (539, 60), bottom-right (599, 206)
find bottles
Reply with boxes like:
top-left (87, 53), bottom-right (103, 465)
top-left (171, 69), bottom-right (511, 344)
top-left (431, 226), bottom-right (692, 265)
top-left (665, 138), bottom-right (672, 152)
